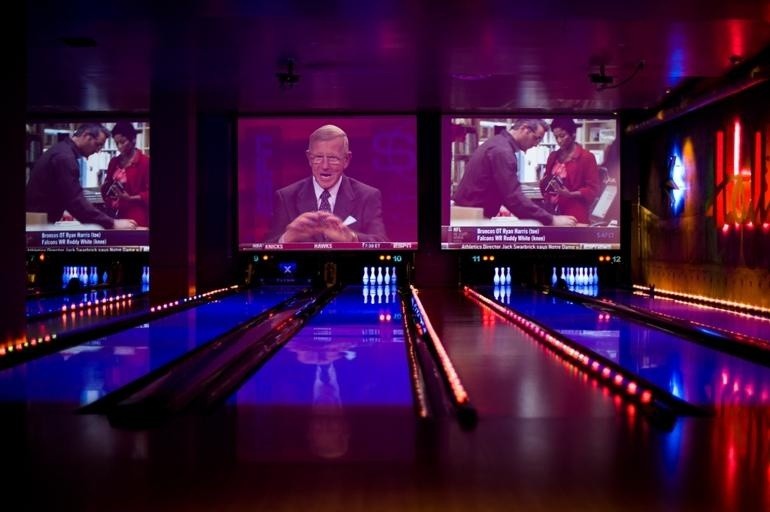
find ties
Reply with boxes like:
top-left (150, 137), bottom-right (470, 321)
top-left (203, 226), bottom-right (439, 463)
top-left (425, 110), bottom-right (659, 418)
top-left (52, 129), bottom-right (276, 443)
top-left (319, 190), bottom-right (331, 242)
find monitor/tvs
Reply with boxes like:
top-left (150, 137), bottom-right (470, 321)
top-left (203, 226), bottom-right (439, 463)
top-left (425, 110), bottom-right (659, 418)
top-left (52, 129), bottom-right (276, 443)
top-left (229, 110), bottom-right (433, 266)
top-left (432, 110), bottom-right (632, 271)
top-left (20, 113), bottom-right (159, 265)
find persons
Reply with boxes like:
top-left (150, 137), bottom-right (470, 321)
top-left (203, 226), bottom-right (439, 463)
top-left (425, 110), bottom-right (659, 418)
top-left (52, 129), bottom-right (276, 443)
top-left (98, 122), bottom-right (149, 231)
top-left (454, 119), bottom-right (577, 228)
top-left (536, 118), bottom-right (603, 222)
top-left (244, 270), bottom-right (418, 465)
top-left (261, 124), bottom-right (390, 243)
top-left (26, 123), bottom-right (139, 232)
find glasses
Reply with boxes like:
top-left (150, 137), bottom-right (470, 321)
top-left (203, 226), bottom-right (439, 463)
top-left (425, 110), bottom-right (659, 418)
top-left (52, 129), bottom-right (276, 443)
top-left (310, 152), bottom-right (347, 165)
